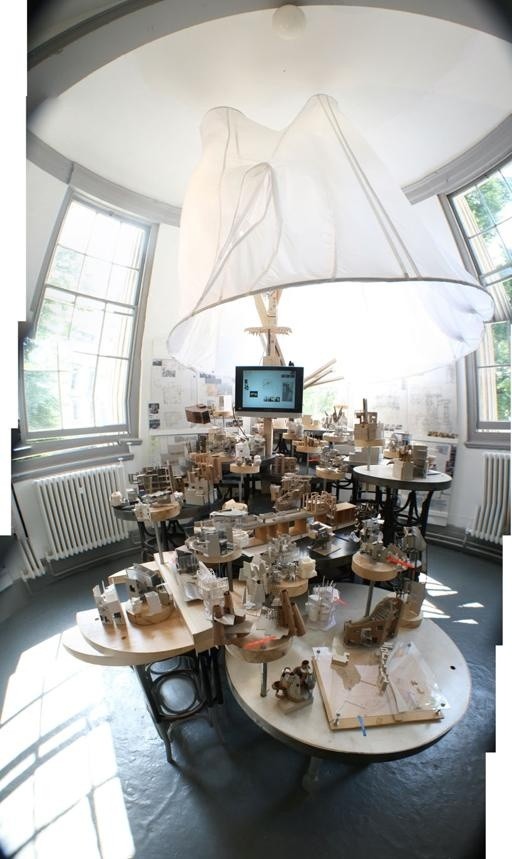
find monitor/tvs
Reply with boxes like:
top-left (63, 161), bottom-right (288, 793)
top-left (235, 366), bottom-right (304, 418)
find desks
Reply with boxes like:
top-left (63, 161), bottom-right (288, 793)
top-left (62, 432), bottom-right (471, 791)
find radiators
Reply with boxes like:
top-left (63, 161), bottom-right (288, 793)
top-left (11, 453), bottom-right (143, 597)
top-left (462, 452), bottom-right (511, 560)
